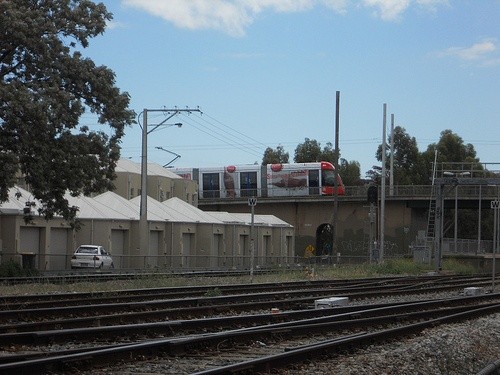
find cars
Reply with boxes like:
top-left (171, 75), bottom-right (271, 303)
top-left (70, 244), bottom-right (115, 271)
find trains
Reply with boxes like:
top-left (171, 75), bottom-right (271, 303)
top-left (165, 160), bottom-right (344, 201)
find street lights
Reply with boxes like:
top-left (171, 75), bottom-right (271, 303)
top-left (140, 122), bottom-right (184, 218)
